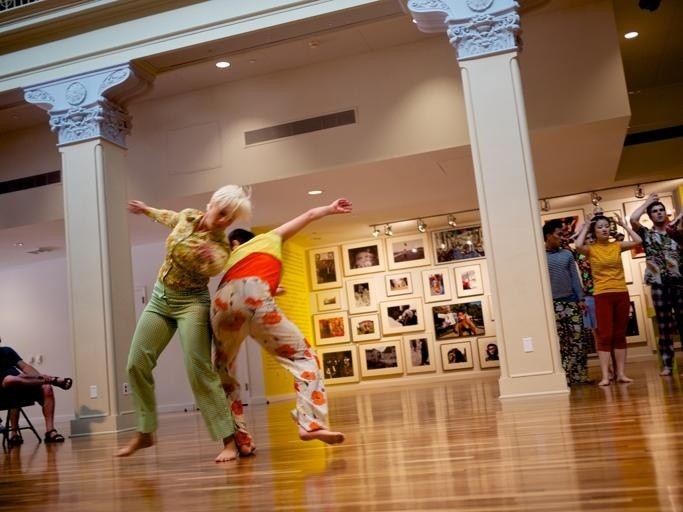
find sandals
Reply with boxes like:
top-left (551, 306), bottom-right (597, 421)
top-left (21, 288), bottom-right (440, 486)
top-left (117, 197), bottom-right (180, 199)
top-left (52, 376), bottom-right (72, 390)
top-left (44, 429), bottom-right (64, 442)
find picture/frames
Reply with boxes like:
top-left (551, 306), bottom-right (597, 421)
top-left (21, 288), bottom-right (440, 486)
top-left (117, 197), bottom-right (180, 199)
top-left (303, 193), bottom-right (677, 390)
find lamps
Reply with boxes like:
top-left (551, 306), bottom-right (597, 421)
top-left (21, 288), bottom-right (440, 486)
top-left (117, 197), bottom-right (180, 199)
top-left (366, 185), bottom-right (654, 237)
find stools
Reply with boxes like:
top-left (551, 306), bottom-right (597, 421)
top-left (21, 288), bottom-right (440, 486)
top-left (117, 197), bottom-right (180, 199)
top-left (0, 402), bottom-right (43, 449)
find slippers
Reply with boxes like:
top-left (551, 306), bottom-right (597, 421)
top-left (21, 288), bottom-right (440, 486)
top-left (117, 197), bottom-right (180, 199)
top-left (7, 434), bottom-right (23, 445)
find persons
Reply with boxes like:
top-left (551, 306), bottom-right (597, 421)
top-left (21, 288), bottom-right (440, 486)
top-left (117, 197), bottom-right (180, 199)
top-left (112, 182), bottom-right (252, 463)
top-left (9, 407), bottom-right (22, 443)
top-left (540, 192), bottom-right (681, 386)
top-left (210, 197), bottom-right (352, 457)
top-left (0, 337), bottom-right (72, 443)
top-left (312, 223), bottom-right (499, 379)
